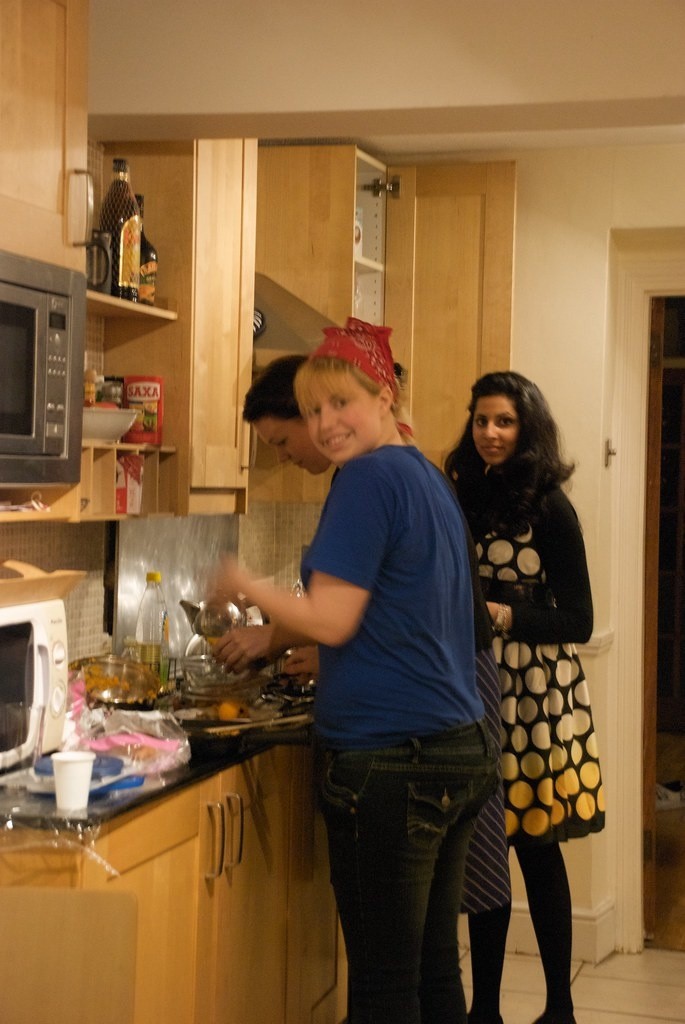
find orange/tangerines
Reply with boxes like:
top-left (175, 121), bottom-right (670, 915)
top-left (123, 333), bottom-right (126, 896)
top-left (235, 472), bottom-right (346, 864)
top-left (218, 700), bottom-right (241, 719)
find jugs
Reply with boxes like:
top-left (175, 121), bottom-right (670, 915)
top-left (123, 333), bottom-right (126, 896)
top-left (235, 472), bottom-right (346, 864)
top-left (178, 588), bottom-right (246, 638)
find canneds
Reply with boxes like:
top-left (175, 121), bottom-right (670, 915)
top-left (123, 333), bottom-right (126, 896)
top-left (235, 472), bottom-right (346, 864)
top-left (121, 375), bottom-right (164, 448)
top-left (95, 375), bottom-right (124, 407)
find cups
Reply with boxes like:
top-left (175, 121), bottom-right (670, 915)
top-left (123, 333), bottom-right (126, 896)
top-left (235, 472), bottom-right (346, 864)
top-left (51, 751), bottom-right (97, 810)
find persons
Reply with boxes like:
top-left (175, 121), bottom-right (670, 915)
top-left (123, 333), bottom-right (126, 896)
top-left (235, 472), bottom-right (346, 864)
top-left (443, 371), bottom-right (605, 1024)
top-left (216, 319), bottom-right (501, 1024)
top-left (219, 354), bottom-right (514, 1024)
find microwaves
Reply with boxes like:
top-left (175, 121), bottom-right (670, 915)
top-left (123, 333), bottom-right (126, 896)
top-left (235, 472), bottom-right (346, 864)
top-left (0, 249), bottom-right (86, 487)
top-left (0, 598), bottom-right (70, 770)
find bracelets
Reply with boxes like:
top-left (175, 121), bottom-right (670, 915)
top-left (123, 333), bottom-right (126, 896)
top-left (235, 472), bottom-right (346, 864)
top-left (494, 603), bottom-right (512, 634)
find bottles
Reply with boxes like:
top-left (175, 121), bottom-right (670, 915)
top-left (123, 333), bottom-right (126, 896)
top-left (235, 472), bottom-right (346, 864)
top-left (133, 194), bottom-right (159, 306)
top-left (96, 157), bottom-right (143, 305)
top-left (135, 572), bottom-right (170, 694)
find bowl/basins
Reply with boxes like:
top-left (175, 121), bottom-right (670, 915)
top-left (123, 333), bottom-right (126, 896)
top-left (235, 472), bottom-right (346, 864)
top-left (82, 407), bottom-right (139, 444)
top-left (177, 653), bottom-right (262, 702)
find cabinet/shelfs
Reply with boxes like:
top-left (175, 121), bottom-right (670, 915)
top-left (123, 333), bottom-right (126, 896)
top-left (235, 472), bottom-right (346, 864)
top-left (0, 751), bottom-right (347, 1024)
top-left (0, 0), bottom-right (519, 525)
